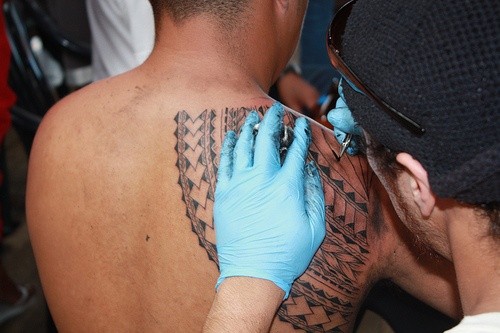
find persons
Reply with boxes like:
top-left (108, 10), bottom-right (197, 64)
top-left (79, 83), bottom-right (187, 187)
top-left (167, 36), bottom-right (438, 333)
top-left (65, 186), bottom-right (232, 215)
top-left (85, 0), bottom-right (319, 115)
top-left (0, 0), bottom-right (27, 320)
top-left (200, 0), bottom-right (500, 333)
top-left (25, 1), bottom-right (463, 333)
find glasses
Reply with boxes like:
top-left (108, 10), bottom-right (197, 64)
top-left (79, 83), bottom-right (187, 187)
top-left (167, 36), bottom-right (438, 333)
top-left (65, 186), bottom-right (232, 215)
top-left (326, 0), bottom-right (426, 139)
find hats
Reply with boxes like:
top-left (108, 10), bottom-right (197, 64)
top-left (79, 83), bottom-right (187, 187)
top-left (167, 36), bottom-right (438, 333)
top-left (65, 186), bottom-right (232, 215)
top-left (339, 0), bottom-right (500, 202)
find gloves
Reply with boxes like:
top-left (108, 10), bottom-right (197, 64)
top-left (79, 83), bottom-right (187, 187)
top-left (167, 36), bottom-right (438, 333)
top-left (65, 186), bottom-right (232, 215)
top-left (213, 101), bottom-right (326, 301)
top-left (326, 96), bottom-right (363, 155)
